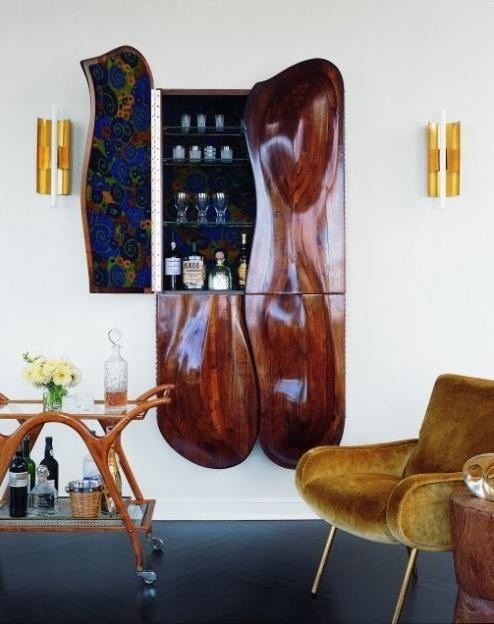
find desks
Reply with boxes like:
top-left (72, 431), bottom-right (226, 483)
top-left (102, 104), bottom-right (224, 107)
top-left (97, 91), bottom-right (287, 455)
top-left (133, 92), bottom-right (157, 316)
top-left (447, 489), bottom-right (494, 624)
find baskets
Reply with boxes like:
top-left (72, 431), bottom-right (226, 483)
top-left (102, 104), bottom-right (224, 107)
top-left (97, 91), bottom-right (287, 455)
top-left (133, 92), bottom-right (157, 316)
top-left (68, 479), bottom-right (104, 522)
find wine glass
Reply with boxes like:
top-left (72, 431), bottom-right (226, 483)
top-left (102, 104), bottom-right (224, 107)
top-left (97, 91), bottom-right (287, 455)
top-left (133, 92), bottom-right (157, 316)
top-left (173, 192), bottom-right (229, 224)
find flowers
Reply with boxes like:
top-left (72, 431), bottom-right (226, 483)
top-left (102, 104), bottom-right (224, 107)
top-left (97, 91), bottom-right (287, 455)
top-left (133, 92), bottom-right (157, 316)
top-left (19, 348), bottom-right (80, 405)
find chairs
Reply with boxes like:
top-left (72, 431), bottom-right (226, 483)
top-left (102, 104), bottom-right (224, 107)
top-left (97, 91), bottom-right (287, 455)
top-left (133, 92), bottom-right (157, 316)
top-left (295, 371), bottom-right (493, 623)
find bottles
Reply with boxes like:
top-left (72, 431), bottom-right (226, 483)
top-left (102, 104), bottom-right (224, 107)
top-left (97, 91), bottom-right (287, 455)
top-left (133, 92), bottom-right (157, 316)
top-left (104, 327), bottom-right (129, 414)
top-left (165, 230), bottom-right (250, 290)
top-left (83, 426), bottom-right (121, 513)
top-left (173, 114), bottom-right (233, 163)
top-left (10, 436), bottom-right (58, 518)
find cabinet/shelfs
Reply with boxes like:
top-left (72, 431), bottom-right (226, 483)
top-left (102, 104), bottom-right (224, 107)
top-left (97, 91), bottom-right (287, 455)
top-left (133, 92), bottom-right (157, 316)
top-left (150, 87), bottom-right (251, 296)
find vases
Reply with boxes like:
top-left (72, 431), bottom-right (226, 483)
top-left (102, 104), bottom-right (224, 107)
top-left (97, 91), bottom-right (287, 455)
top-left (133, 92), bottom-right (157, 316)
top-left (41, 390), bottom-right (62, 413)
